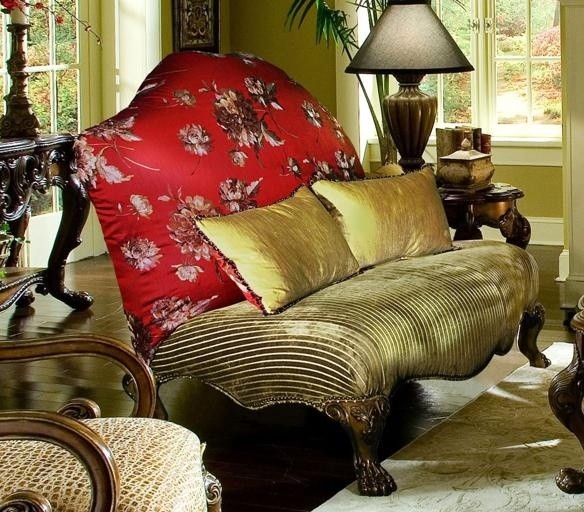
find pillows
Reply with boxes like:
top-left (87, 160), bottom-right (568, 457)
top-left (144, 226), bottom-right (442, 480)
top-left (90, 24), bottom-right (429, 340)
top-left (196, 186), bottom-right (362, 313)
top-left (309, 167), bottom-right (455, 266)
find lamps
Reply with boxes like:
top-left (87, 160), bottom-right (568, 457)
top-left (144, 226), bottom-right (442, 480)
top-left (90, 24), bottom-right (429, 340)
top-left (344, 0), bottom-right (474, 173)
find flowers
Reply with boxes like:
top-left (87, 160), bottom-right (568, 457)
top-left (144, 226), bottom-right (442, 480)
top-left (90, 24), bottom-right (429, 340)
top-left (1, 0), bottom-right (103, 44)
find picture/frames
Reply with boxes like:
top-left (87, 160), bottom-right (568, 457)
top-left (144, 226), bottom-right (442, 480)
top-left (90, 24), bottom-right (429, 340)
top-left (172, 0), bottom-right (219, 54)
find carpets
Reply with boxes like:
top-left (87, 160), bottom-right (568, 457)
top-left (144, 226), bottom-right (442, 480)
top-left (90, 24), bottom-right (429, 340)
top-left (295, 337), bottom-right (584, 512)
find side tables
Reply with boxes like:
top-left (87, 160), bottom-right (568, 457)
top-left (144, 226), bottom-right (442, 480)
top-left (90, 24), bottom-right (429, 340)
top-left (1, 133), bottom-right (91, 319)
top-left (437, 182), bottom-right (530, 249)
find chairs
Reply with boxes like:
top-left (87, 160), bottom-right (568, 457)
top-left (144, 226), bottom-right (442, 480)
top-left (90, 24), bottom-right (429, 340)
top-left (1, 332), bottom-right (223, 511)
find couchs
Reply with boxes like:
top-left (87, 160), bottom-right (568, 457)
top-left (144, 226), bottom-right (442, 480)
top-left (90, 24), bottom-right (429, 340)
top-left (67, 48), bottom-right (550, 491)
